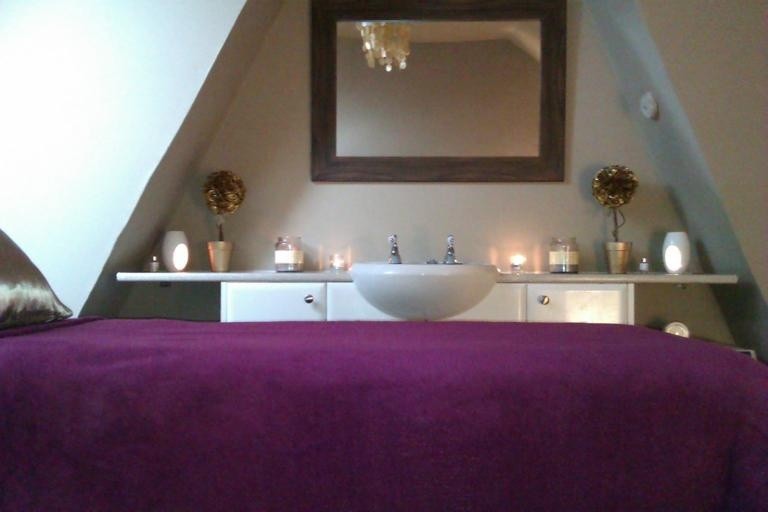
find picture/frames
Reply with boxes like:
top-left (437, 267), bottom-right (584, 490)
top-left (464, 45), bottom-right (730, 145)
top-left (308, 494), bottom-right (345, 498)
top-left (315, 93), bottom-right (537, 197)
top-left (308, 0), bottom-right (568, 184)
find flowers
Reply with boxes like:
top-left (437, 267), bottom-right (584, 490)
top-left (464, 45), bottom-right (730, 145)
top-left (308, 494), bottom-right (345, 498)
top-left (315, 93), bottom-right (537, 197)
top-left (591, 165), bottom-right (638, 242)
top-left (201, 170), bottom-right (245, 241)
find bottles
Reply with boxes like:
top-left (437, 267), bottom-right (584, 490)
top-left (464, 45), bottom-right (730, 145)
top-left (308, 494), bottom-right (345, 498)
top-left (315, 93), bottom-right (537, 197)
top-left (160, 230), bottom-right (190, 270)
top-left (275, 236), bottom-right (304, 273)
top-left (661, 230), bottom-right (690, 275)
top-left (547, 235), bottom-right (580, 273)
top-left (150, 255), bottom-right (160, 274)
top-left (445, 234), bottom-right (455, 262)
top-left (636, 258), bottom-right (650, 272)
top-left (385, 239), bottom-right (403, 265)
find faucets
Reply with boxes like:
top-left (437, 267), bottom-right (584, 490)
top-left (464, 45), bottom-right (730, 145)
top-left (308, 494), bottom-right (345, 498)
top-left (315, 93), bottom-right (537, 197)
top-left (387, 233), bottom-right (402, 264)
top-left (445, 234), bottom-right (457, 265)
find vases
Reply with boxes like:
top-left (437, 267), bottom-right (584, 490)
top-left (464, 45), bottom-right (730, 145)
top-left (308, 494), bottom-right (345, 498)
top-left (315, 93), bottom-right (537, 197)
top-left (207, 239), bottom-right (233, 272)
top-left (604, 241), bottom-right (632, 275)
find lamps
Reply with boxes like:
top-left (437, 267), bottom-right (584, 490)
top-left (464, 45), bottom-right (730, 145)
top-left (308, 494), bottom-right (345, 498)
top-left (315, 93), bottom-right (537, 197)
top-left (357, 19), bottom-right (413, 74)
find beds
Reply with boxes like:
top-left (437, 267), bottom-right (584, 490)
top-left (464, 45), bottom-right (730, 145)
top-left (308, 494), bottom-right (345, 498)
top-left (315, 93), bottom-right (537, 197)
top-left (0, 315), bottom-right (767, 512)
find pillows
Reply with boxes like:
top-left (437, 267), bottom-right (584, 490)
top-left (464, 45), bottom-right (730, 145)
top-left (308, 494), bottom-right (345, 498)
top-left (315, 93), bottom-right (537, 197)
top-left (0, 224), bottom-right (70, 329)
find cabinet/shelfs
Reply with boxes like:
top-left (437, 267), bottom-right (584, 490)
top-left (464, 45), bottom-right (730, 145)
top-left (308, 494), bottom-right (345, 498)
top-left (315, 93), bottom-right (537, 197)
top-left (525, 284), bottom-right (636, 327)
top-left (219, 281), bottom-right (327, 323)
top-left (326, 283), bottom-right (524, 321)
